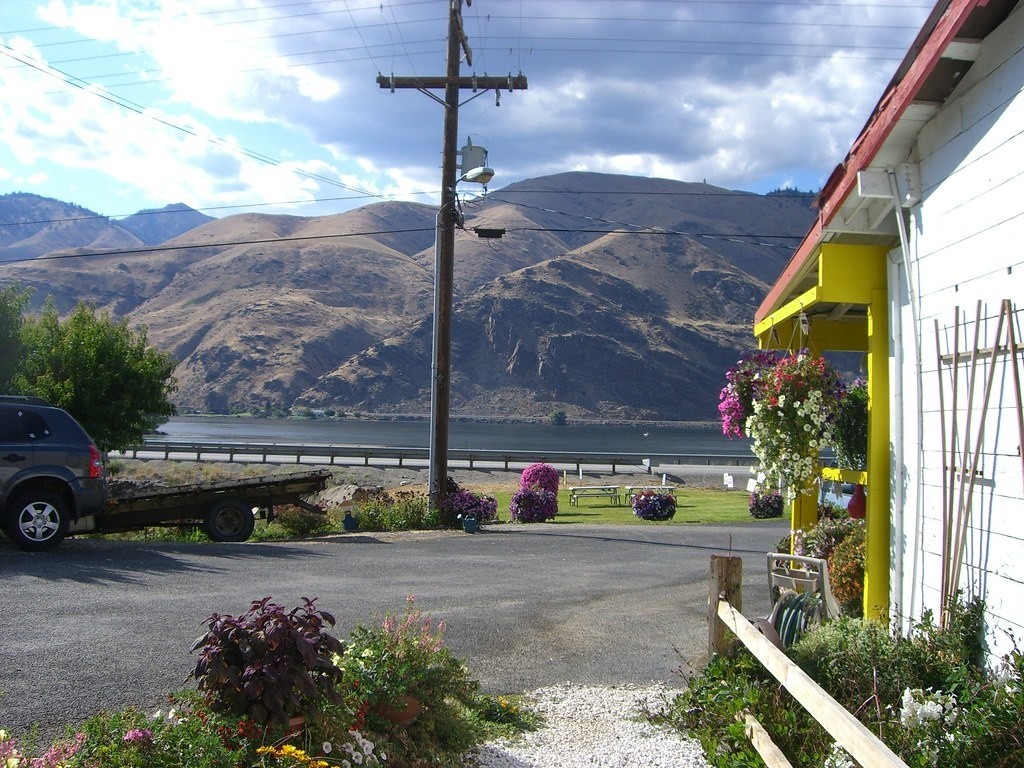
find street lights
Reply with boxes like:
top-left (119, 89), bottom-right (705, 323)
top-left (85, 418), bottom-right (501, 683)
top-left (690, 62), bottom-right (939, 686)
top-left (428, 166), bottom-right (495, 510)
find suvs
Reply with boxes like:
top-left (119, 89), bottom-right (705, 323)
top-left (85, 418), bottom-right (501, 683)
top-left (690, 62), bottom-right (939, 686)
top-left (0, 395), bottom-right (107, 553)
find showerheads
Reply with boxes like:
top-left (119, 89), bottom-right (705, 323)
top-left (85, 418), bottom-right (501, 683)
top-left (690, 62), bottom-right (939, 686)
top-left (456, 513), bottom-right (463, 520)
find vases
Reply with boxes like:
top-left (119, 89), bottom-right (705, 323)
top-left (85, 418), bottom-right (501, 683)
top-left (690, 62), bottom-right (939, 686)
top-left (376, 688), bottom-right (434, 727)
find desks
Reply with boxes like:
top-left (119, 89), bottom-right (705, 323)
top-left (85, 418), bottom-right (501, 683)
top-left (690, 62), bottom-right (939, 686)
top-left (569, 486), bottom-right (620, 507)
top-left (625, 485), bottom-right (677, 498)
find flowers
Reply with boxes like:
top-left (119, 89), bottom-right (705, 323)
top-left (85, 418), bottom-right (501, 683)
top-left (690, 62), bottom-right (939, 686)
top-left (335, 598), bottom-right (474, 717)
top-left (717, 348), bottom-right (867, 499)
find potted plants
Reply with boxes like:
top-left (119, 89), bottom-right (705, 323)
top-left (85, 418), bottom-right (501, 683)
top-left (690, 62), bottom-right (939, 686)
top-left (184, 595), bottom-right (345, 744)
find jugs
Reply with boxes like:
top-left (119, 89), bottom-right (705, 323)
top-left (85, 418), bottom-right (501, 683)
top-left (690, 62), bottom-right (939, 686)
top-left (457, 512), bottom-right (477, 533)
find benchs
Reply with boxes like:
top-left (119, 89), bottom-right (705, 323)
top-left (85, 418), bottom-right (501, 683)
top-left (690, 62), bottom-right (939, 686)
top-left (625, 493), bottom-right (679, 505)
top-left (569, 492), bottom-right (621, 508)
